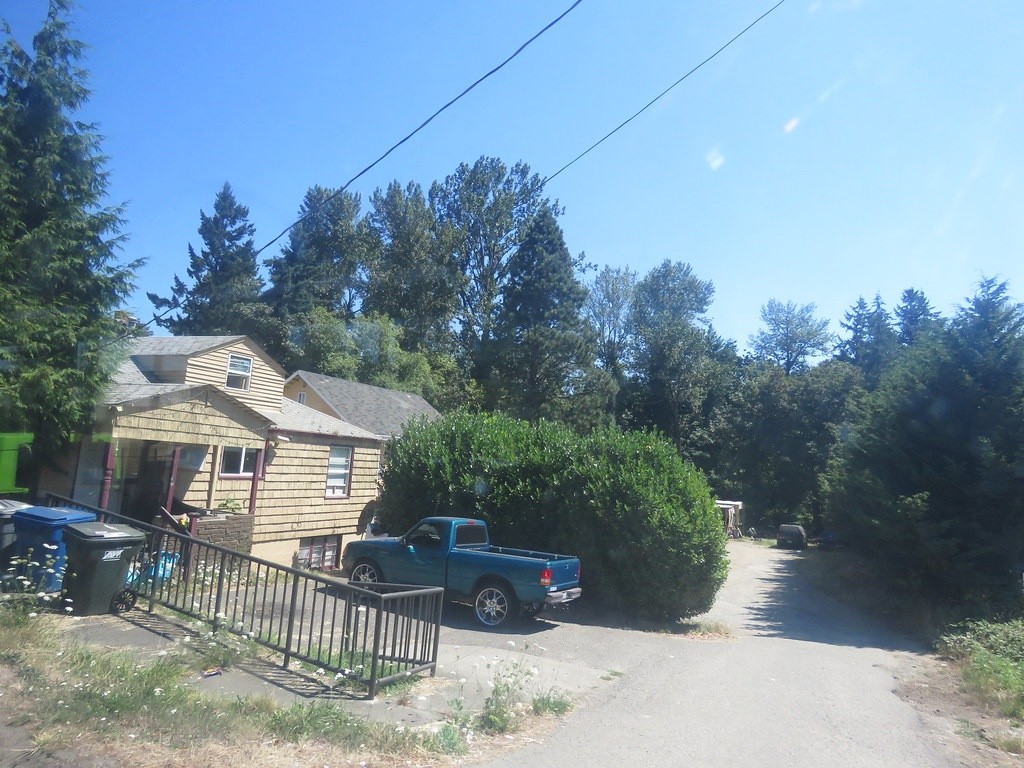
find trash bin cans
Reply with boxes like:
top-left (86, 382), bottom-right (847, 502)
top-left (60, 523), bottom-right (152, 617)
top-left (0, 500), bottom-right (32, 570)
top-left (11, 506), bottom-right (98, 594)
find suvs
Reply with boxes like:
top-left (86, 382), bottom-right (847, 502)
top-left (777, 524), bottom-right (808, 548)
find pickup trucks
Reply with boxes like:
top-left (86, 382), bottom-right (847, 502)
top-left (341, 517), bottom-right (583, 628)
